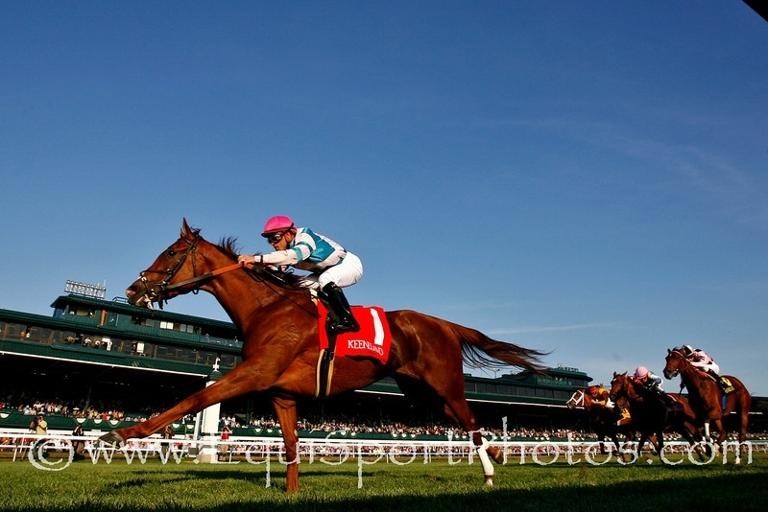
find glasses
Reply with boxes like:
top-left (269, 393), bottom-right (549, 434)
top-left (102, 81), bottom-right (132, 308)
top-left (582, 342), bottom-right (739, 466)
top-left (268, 233), bottom-right (287, 244)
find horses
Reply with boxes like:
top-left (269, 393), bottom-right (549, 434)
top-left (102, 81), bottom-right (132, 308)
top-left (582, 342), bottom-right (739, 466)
top-left (662, 346), bottom-right (768, 465)
top-left (564, 387), bottom-right (659, 465)
top-left (98, 216), bottom-right (556, 495)
top-left (609, 371), bottom-right (718, 463)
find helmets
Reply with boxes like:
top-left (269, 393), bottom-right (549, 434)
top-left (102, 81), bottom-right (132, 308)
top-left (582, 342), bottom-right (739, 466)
top-left (682, 344), bottom-right (694, 357)
top-left (261, 217), bottom-right (296, 236)
top-left (635, 367), bottom-right (647, 379)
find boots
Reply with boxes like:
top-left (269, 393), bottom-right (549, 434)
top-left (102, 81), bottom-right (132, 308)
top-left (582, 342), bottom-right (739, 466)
top-left (323, 281), bottom-right (359, 333)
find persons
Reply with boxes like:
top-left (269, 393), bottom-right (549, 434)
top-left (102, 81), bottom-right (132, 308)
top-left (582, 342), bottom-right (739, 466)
top-left (0, 327), bottom-right (768, 460)
top-left (238, 216), bottom-right (363, 335)
